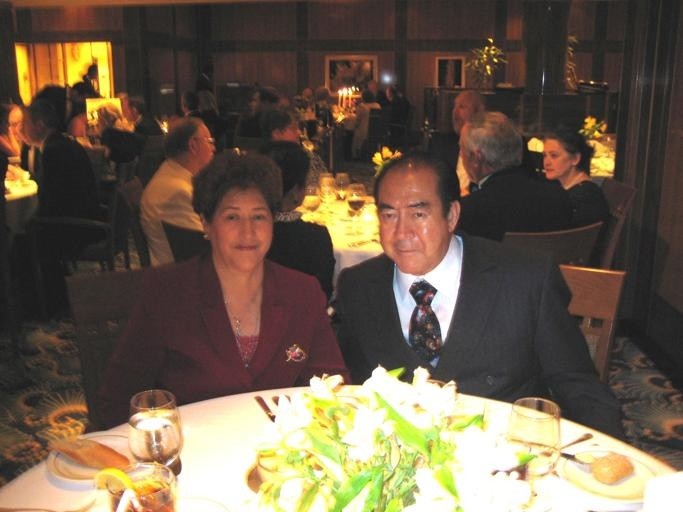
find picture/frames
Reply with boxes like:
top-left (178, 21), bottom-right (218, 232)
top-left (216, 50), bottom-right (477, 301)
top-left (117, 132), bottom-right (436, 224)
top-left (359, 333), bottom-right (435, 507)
top-left (325, 54), bottom-right (378, 98)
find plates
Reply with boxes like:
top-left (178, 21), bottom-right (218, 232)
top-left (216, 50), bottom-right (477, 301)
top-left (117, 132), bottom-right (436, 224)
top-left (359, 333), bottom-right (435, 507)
top-left (46, 431), bottom-right (141, 483)
top-left (563, 452), bottom-right (658, 500)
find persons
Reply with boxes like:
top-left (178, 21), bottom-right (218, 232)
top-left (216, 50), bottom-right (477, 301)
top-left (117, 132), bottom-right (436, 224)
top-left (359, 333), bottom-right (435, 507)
top-left (100, 153), bottom-right (347, 430)
top-left (2, 65), bottom-right (609, 305)
top-left (334, 150), bottom-right (627, 444)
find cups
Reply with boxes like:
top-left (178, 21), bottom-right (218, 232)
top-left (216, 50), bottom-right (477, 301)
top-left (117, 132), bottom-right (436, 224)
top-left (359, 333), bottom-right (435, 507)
top-left (510, 396), bottom-right (562, 478)
top-left (104, 462), bottom-right (174, 512)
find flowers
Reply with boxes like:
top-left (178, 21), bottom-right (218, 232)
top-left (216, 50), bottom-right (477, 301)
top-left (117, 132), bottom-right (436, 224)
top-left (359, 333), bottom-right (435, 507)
top-left (464, 37), bottom-right (508, 75)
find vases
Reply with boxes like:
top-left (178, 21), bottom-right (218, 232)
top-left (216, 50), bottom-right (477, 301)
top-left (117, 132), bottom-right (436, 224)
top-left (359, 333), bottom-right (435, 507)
top-left (482, 71), bottom-right (494, 89)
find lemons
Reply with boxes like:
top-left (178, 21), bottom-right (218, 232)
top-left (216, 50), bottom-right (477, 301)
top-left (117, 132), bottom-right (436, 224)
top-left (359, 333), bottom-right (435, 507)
top-left (95, 467), bottom-right (133, 490)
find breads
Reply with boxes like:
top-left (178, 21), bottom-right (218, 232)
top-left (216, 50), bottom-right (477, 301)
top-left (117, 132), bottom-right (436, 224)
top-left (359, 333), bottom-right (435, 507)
top-left (47, 438), bottom-right (130, 470)
top-left (591, 453), bottom-right (634, 484)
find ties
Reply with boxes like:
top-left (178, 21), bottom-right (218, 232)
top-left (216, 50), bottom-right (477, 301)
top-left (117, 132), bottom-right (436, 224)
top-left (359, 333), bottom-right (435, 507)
top-left (408, 278), bottom-right (443, 362)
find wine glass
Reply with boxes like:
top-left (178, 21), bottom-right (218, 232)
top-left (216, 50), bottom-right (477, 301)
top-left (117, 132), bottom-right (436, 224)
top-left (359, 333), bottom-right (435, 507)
top-left (126, 390), bottom-right (180, 480)
top-left (301, 173), bottom-right (378, 248)
